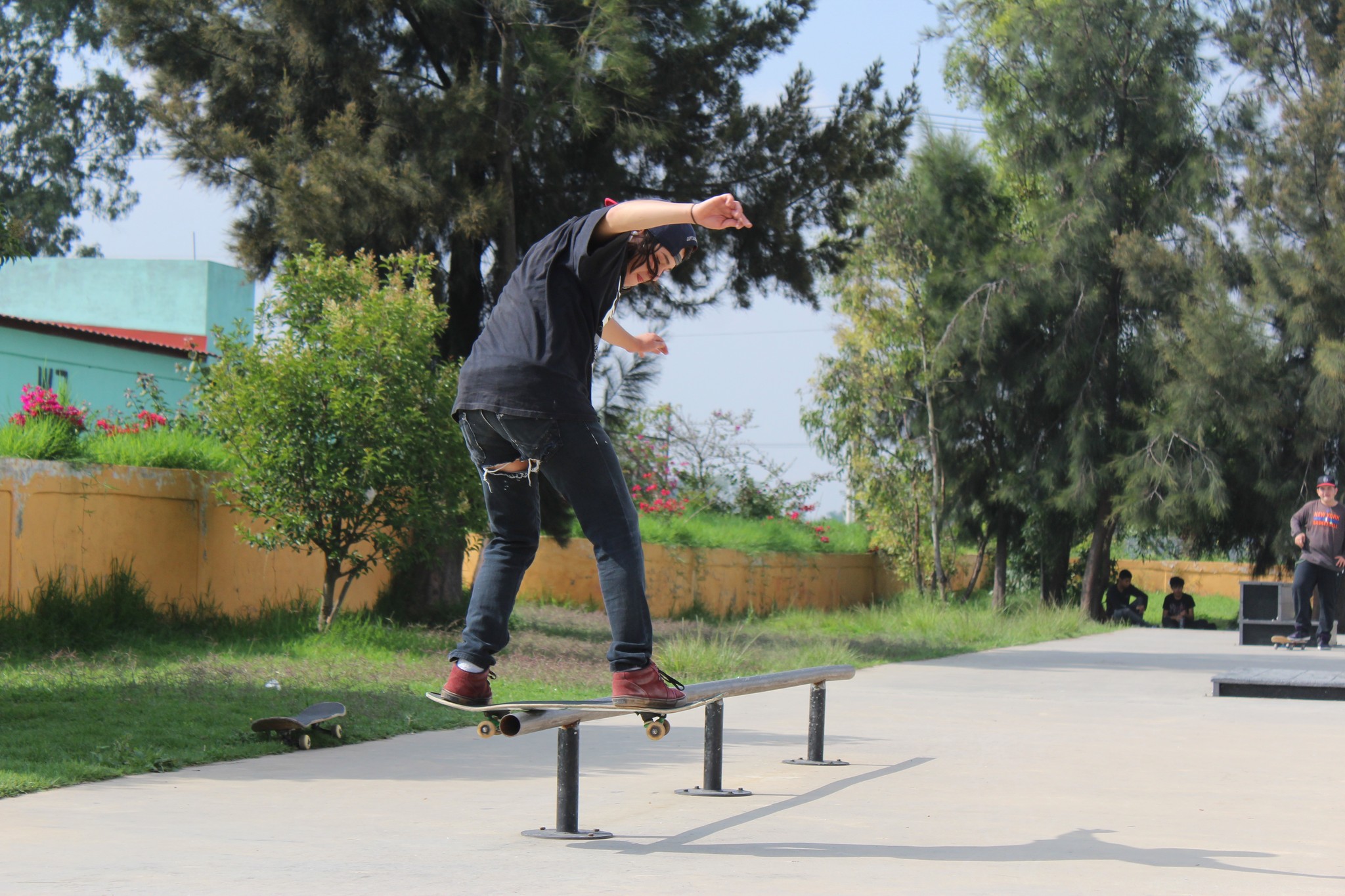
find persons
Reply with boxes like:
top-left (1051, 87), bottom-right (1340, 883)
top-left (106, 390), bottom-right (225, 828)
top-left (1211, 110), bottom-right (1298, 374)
top-left (1287, 475), bottom-right (1345, 650)
top-left (1106, 570), bottom-right (1158, 627)
top-left (1161, 577), bottom-right (1208, 629)
top-left (441, 193), bottom-right (753, 712)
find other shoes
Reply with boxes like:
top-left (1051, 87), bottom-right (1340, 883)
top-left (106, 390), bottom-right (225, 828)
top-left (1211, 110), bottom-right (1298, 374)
top-left (1287, 629), bottom-right (1311, 642)
top-left (1318, 637), bottom-right (1332, 650)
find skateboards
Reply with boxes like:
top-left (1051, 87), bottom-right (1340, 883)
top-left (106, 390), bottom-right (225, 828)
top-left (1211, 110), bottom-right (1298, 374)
top-left (1271, 636), bottom-right (1311, 650)
top-left (426, 691), bottom-right (726, 741)
top-left (251, 702), bottom-right (347, 750)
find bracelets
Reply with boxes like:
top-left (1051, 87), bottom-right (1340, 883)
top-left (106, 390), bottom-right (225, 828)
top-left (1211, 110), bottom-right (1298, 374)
top-left (691, 204), bottom-right (699, 225)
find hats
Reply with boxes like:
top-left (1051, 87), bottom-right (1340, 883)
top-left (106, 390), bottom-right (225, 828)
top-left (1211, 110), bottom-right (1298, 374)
top-left (1316, 475), bottom-right (1336, 488)
top-left (605, 197), bottom-right (699, 267)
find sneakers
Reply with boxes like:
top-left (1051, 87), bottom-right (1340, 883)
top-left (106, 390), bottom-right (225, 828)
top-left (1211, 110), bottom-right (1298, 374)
top-left (612, 660), bottom-right (687, 708)
top-left (441, 660), bottom-right (497, 706)
